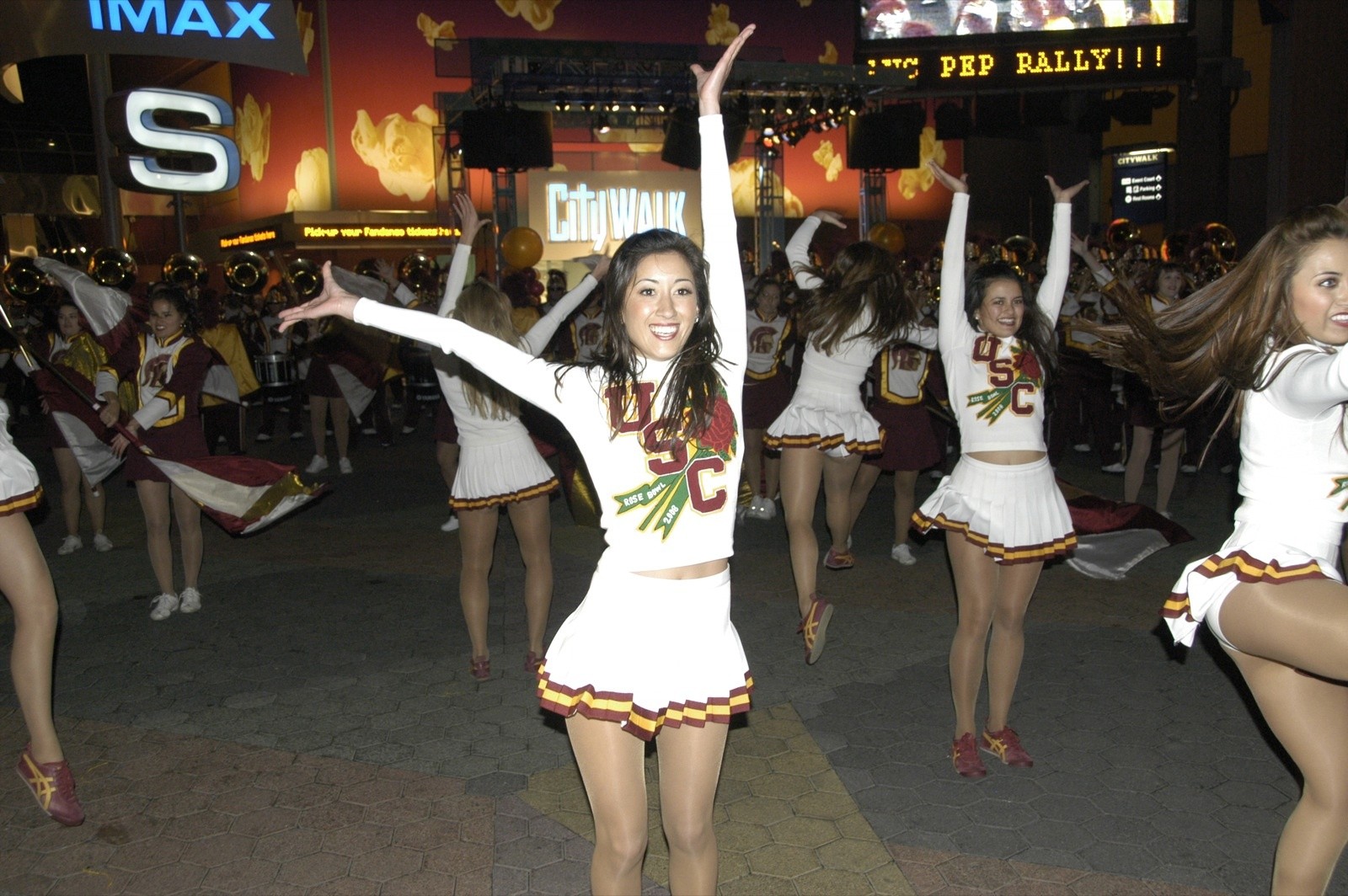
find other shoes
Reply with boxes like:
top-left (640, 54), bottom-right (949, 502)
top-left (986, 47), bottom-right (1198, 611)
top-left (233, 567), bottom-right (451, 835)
top-left (756, 497), bottom-right (776, 520)
top-left (931, 470), bottom-right (943, 478)
top-left (1161, 511), bottom-right (1171, 519)
top-left (525, 652), bottom-right (544, 671)
top-left (746, 496), bottom-right (762, 517)
top-left (441, 515), bottom-right (459, 531)
top-left (1102, 462), bottom-right (1127, 472)
top-left (1221, 465), bottom-right (1233, 473)
top-left (1075, 444), bottom-right (1090, 451)
top-left (1182, 465), bottom-right (1197, 473)
top-left (468, 658), bottom-right (492, 683)
top-left (216, 403), bottom-right (437, 444)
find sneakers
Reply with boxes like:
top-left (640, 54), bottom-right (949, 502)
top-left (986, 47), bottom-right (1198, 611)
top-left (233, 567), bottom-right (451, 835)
top-left (180, 587), bottom-right (201, 613)
top-left (93, 534), bottom-right (113, 552)
top-left (58, 535), bottom-right (83, 555)
top-left (981, 725), bottom-right (1035, 769)
top-left (14, 743), bottom-right (86, 826)
top-left (891, 544), bottom-right (918, 565)
top-left (339, 457), bottom-right (352, 475)
top-left (305, 454), bottom-right (329, 473)
top-left (797, 593), bottom-right (834, 664)
top-left (826, 549), bottom-right (855, 569)
top-left (951, 733), bottom-right (986, 777)
top-left (151, 592), bottom-right (180, 619)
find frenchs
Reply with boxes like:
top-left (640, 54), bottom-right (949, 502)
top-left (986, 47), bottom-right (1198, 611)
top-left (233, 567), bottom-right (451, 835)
top-left (281, 258), bottom-right (324, 303)
top-left (1085, 218), bottom-right (1237, 288)
top-left (926, 240), bottom-right (944, 276)
top-left (161, 252), bottom-right (211, 299)
top-left (353, 259), bottom-right (389, 285)
top-left (88, 246), bottom-right (138, 294)
top-left (223, 249), bottom-right (270, 296)
top-left (399, 253), bottom-right (439, 298)
top-left (999, 235), bottom-right (1038, 276)
top-left (1, 256), bottom-right (49, 305)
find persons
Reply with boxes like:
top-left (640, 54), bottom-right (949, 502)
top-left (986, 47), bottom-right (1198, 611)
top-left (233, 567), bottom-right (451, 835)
top-left (0, 395), bottom-right (85, 827)
top-left (0, 232), bottom-right (1348, 563)
top-left (764, 205), bottom-right (940, 664)
top-left (277, 25), bottom-right (760, 896)
top-left (97, 291), bottom-right (239, 621)
top-left (926, 160), bottom-right (1089, 781)
top-left (1162, 204), bottom-right (1348, 896)
top-left (432, 189), bottom-right (610, 679)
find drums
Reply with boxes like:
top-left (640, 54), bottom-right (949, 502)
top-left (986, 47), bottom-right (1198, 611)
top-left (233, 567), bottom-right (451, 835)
top-left (408, 351), bottom-right (443, 402)
top-left (250, 354), bottom-right (300, 388)
